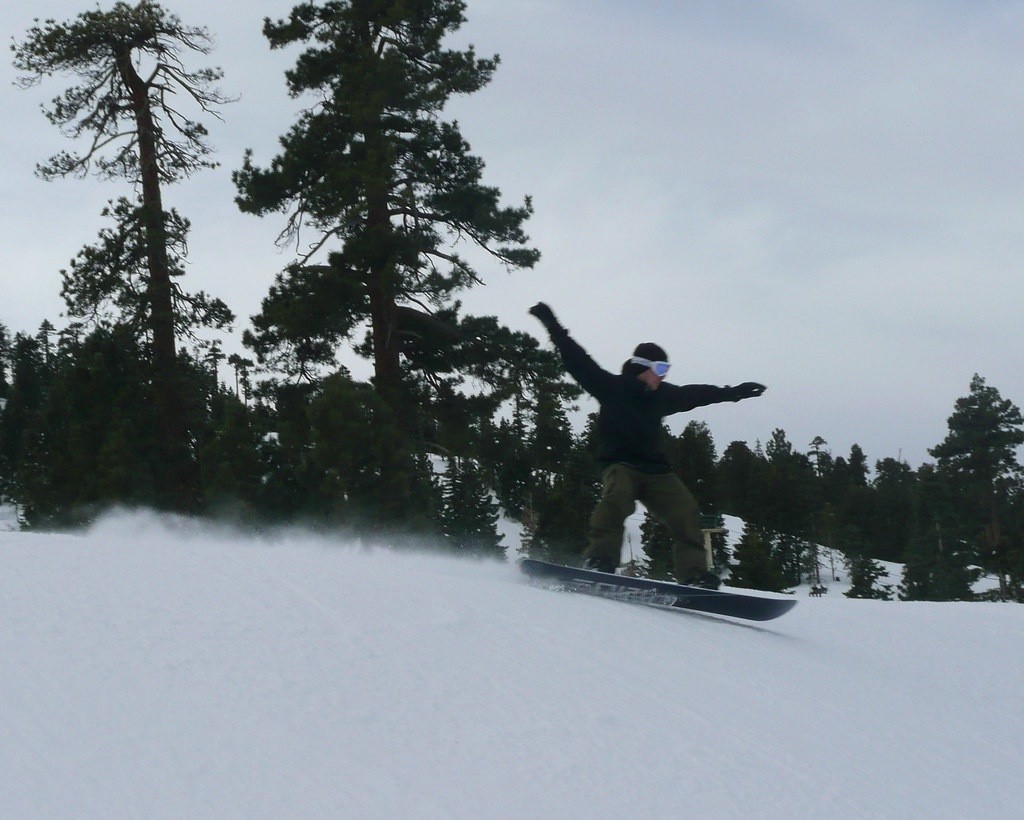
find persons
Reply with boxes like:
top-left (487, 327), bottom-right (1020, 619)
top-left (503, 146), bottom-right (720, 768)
top-left (530, 300), bottom-right (768, 591)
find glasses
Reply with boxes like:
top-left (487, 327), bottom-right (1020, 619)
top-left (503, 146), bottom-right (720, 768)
top-left (651, 361), bottom-right (672, 377)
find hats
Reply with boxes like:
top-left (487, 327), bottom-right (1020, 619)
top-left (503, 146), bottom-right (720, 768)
top-left (622, 343), bottom-right (668, 374)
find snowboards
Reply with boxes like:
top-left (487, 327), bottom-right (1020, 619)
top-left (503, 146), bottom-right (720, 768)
top-left (520, 559), bottom-right (797, 622)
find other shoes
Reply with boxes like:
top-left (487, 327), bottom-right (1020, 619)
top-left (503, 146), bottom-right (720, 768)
top-left (583, 557), bottom-right (615, 574)
top-left (684, 570), bottom-right (721, 589)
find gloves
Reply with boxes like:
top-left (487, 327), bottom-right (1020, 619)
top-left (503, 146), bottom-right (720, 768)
top-left (530, 302), bottom-right (562, 330)
top-left (732, 382), bottom-right (766, 402)
